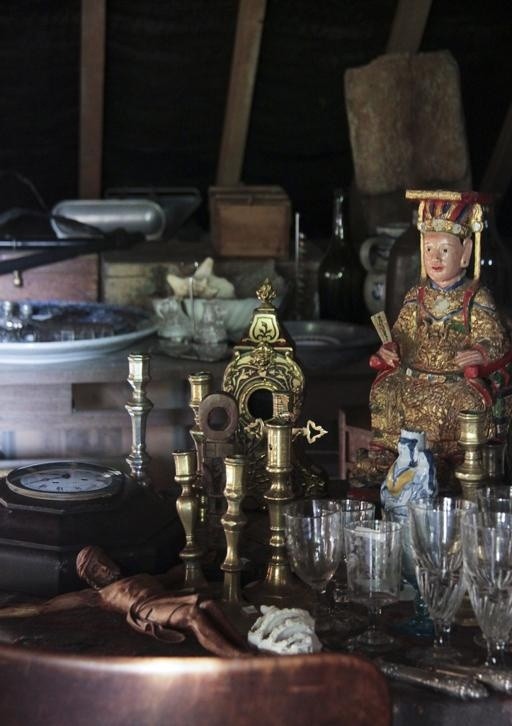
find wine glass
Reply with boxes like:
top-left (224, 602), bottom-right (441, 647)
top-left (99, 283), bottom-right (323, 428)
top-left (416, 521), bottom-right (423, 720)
top-left (280, 496), bottom-right (512, 672)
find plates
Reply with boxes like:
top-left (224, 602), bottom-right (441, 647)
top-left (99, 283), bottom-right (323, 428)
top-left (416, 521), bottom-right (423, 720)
top-left (0, 298), bottom-right (169, 367)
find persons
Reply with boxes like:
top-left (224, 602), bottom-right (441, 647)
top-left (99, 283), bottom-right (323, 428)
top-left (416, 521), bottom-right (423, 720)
top-left (352, 188), bottom-right (510, 481)
top-left (378, 429), bottom-right (437, 523)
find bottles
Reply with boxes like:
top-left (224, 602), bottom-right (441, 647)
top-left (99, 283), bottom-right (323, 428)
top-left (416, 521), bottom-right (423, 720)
top-left (158, 291), bottom-right (228, 363)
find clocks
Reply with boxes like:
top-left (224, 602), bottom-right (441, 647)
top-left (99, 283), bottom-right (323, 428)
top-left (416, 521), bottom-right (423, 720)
top-left (0, 462), bottom-right (187, 598)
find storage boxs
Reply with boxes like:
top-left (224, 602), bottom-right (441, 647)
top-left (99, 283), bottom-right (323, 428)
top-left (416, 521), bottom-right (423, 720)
top-left (1, 184), bottom-right (291, 311)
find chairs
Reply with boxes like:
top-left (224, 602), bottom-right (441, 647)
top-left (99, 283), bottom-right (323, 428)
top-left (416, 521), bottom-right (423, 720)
top-left (0, 648), bottom-right (392, 726)
